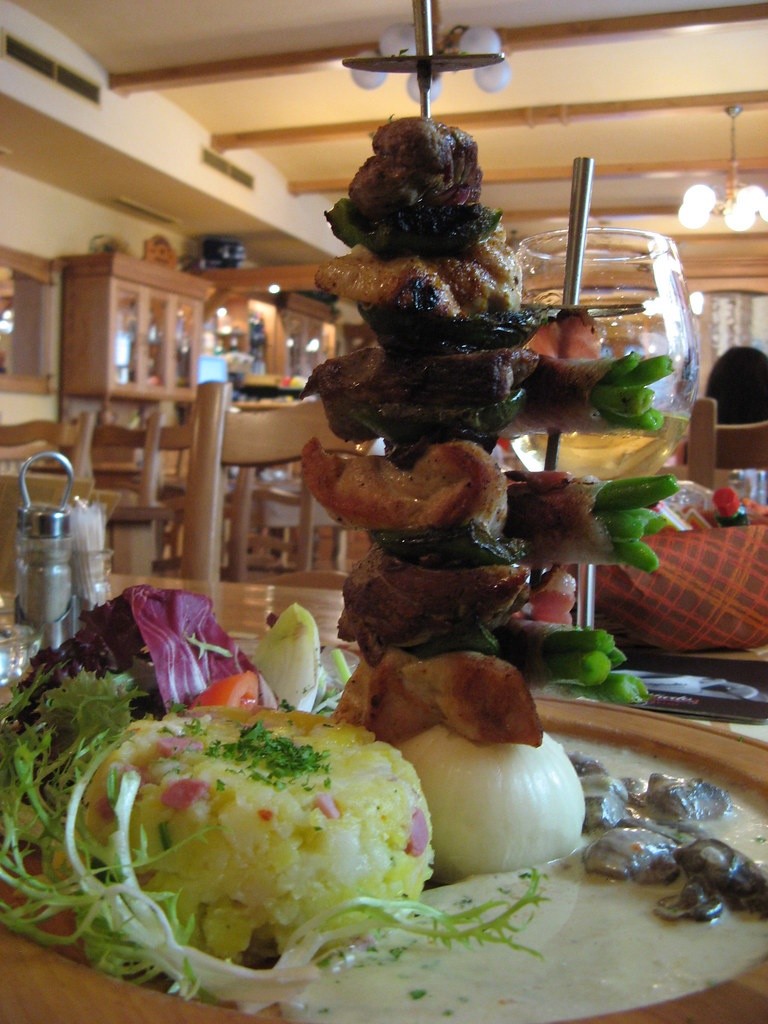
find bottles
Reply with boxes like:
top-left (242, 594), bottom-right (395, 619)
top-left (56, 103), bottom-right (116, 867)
top-left (645, 468), bottom-right (748, 531)
top-left (70, 550), bottom-right (113, 613)
top-left (17, 450), bottom-right (75, 651)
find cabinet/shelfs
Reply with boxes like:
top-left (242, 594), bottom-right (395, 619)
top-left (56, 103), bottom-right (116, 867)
top-left (56, 253), bottom-right (216, 424)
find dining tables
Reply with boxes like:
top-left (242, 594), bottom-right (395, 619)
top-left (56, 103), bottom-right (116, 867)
top-left (1, 575), bottom-right (768, 1024)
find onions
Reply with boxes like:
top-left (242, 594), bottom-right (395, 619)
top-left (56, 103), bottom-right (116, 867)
top-left (399, 713), bottom-right (585, 879)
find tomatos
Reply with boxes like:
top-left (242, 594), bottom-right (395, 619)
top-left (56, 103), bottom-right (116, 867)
top-left (187, 671), bottom-right (260, 711)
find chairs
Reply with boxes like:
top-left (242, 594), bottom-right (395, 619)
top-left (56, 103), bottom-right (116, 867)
top-left (0, 352), bottom-right (768, 581)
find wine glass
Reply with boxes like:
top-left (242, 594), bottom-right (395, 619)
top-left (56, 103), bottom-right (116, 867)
top-left (505, 228), bottom-right (700, 627)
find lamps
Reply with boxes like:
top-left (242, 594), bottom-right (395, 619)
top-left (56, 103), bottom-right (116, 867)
top-left (350, 0), bottom-right (510, 104)
top-left (678, 103), bottom-right (768, 231)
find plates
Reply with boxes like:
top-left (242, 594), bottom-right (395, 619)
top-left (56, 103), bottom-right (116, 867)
top-left (1, 694), bottom-right (768, 1024)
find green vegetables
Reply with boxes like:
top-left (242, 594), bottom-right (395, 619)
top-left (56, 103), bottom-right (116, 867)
top-left (0, 584), bottom-right (551, 1017)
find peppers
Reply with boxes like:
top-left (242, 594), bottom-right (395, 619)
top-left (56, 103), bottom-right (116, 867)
top-left (322, 195), bottom-right (551, 655)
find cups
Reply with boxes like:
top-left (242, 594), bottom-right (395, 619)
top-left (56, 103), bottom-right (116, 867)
top-left (730, 469), bottom-right (766, 505)
